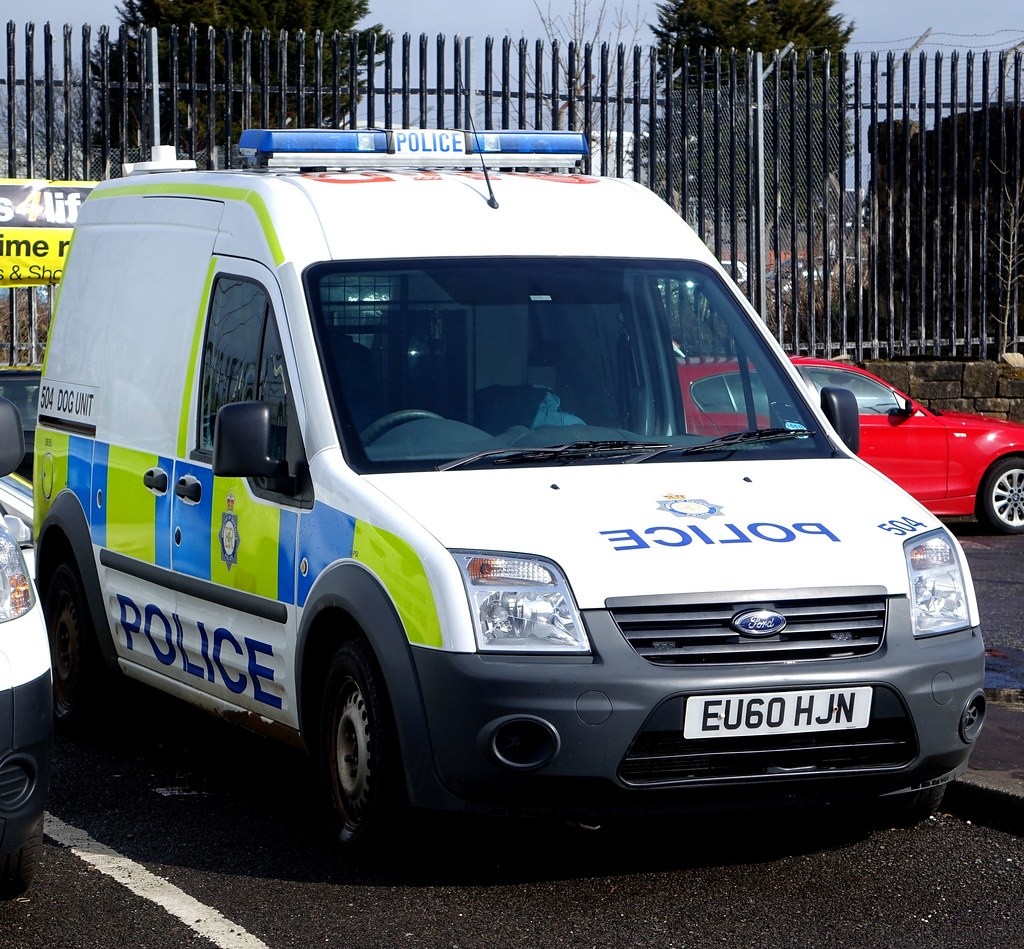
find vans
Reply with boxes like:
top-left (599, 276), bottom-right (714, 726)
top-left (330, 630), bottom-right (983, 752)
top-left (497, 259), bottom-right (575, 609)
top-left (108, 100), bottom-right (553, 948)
top-left (0, 395), bottom-right (54, 904)
top-left (29, 123), bottom-right (988, 863)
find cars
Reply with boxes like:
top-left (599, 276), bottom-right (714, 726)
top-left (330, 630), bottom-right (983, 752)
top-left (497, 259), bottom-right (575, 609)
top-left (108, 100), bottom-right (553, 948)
top-left (673, 356), bottom-right (1024, 536)
top-left (719, 255), bottom-right (870, 297)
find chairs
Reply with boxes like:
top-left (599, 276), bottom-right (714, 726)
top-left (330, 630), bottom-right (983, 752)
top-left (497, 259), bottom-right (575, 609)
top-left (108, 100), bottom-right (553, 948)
top-left (472, 382), bottom-right (563, 436)
